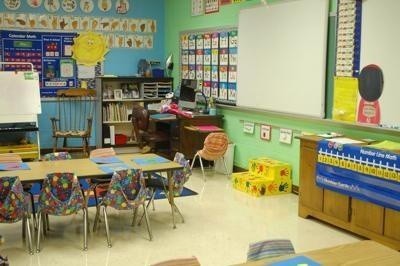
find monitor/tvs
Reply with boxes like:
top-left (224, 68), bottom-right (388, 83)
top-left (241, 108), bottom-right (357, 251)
top-left (178, 78), bottom-right (197, 111)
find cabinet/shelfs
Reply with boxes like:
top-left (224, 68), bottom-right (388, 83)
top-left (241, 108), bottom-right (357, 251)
top-left (294, 130), bottom-right (400, 252)
top-left (148, 104), bottom-right (225, 166)
top-left (95, 73), bottom-right (174, 151)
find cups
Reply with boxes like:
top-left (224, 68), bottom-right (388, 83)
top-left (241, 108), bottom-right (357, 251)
top-left (45, 42), bottom-right (60, 56)
top-left (81, 81), bottom-right (87, 89)
top-left (209, 109), bottom-right (217, 115)
top-left (144, 69), bottom-right (151, 77)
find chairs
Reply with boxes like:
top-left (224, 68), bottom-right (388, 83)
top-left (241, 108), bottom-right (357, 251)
top-left (0, 146), bottom-right (192, 255)
top-left (130, 100), bottom-right (170, 156)
top-left (49, 85), bottom-right (101, 156)
top-left (145, 238), bottom-right (400, 266)
top-left (190, 129), bottom-right (232, 182)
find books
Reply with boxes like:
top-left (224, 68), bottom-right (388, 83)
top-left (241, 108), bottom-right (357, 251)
top-left (103, 102), bottom-right (129, 121)
top-left (152, 113), bottom-right (177, 120)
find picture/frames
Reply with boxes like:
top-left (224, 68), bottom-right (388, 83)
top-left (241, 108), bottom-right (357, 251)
top-left (113, 88), bottom-right (122, 99)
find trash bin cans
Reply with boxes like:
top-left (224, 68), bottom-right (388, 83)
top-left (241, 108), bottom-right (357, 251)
top-left (213, 143), bottom-right (235, 174)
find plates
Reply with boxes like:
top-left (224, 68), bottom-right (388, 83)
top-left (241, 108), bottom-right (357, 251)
top-left (116, 0), bottom-right (129, 11)
top-left (79, 0), bottom-right (94, 12)
top-left (61, 0), bottom-right (77, 12)
top-left (3, 0), bottom-right (21, 10)
top-left (44, 0), bottom-right (60, 12)
top-left (97, 0), bottom-right (112, 12)
top-left (27, 0), bottom-right (42, 7)
